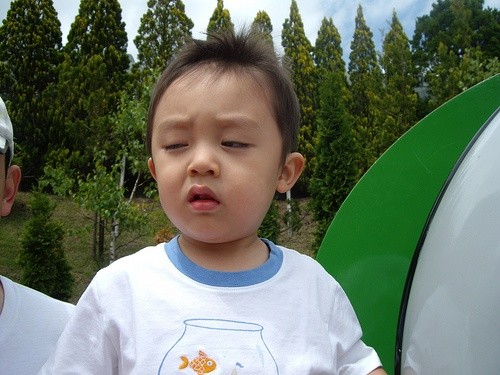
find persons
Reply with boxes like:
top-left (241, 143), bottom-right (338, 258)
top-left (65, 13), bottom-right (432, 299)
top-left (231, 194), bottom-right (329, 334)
top-left (0, 95), bottom-right (75, 375)
top-left (37, 36), bottom-right (385, 375)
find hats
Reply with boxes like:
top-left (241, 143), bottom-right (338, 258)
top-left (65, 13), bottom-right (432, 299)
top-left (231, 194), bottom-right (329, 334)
top-left (0, 98), bottom-right (14, 167)
